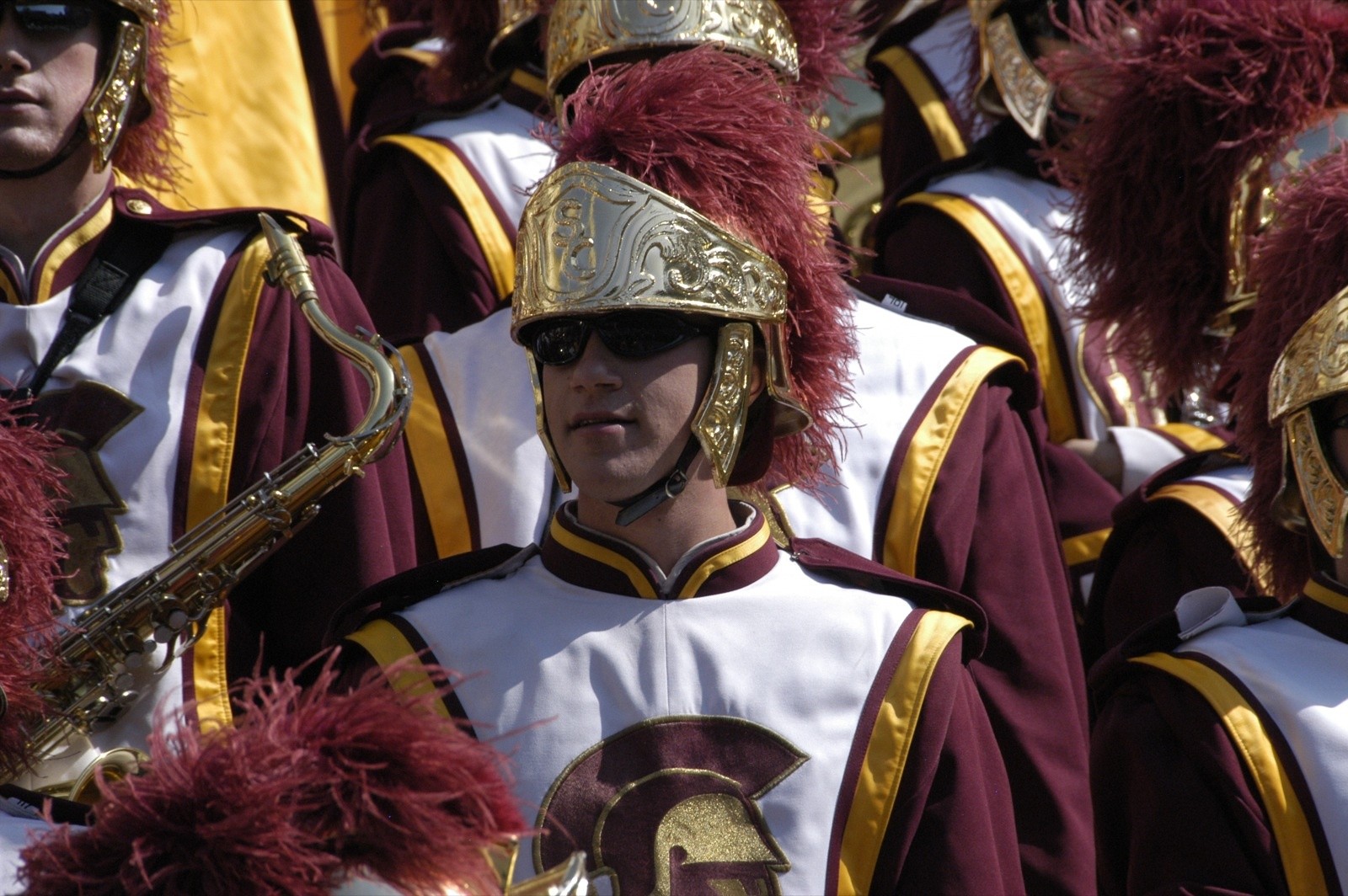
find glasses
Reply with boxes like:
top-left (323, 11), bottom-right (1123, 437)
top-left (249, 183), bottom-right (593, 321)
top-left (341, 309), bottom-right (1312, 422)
top-left (0, 0), bottom-right (113, 42)
top-left (525, 306), bottom-right (723, 368)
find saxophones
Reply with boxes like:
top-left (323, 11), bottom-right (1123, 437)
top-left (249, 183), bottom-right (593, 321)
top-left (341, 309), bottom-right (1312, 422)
top-left (0, 210), bottom-right (413, 823)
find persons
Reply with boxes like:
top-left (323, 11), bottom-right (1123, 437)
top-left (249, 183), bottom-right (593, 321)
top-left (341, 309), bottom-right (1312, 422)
top-left (0, 0), bottom-right (1348, 896)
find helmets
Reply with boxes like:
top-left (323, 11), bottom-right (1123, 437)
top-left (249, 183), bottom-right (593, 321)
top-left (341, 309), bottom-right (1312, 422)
top-left (488, 0), bottom-right (552, 57)
top-left (501, 43), bottom-right (862, 490)
top-left (1224, 144), bottom-right (1348, 603)
top-left (1043, 0), bottom-right (1348, 403)
top-left (0, 0), bottom-right (170, 175)
top-left (543, 0), bottom-right (866, 132)
